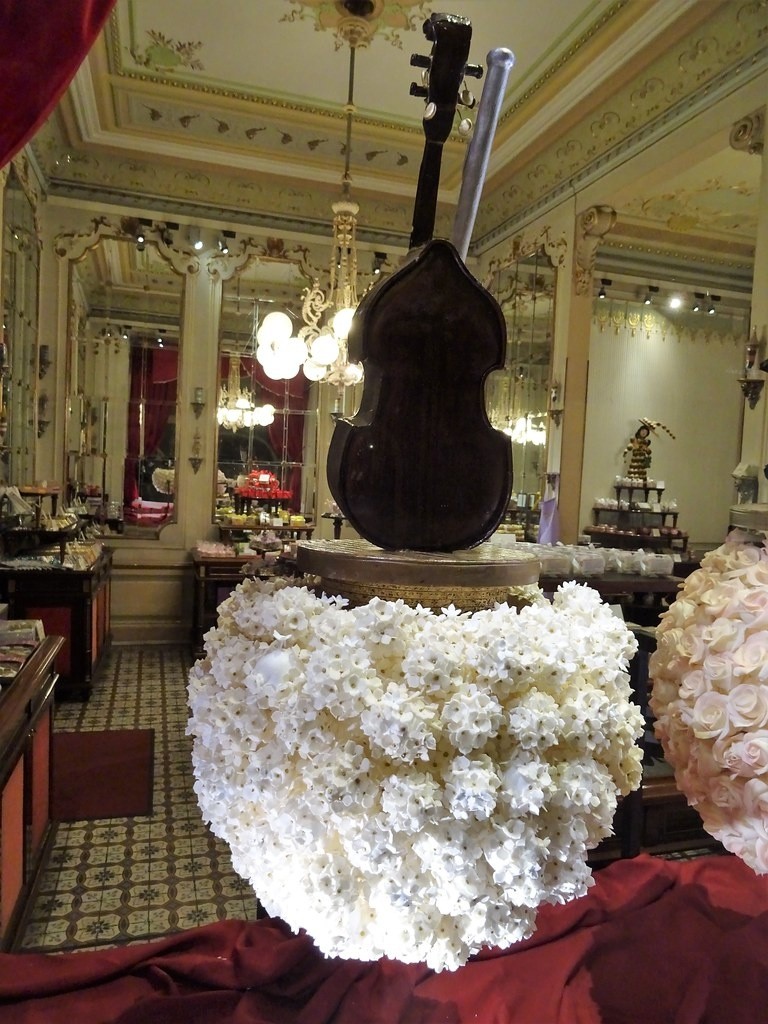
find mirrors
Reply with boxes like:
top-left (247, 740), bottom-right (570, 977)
top-left (63, 235), bottom-right (188, 541)
top-left (0, 155), bottom-right (43, 486)
top-left (478, 242), bottom-right (560, 535)
top-left (213, 234), bottom-right (326, 532)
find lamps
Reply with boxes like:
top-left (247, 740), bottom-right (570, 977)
top-left (156, 328), bottom-right (169, 347)
top-left (705, 295), bottom-right (721, 315)
top-left (254, 0), bottom-right (431, 420)
top-left (105, 322), bottom-right (115, 337)
top-left (643, 287), bottom-right (659, 305)
top-left (736, 322), bottom-right (766, 409)
top-left (217, 274), bottom-right (275, 433)
top-left (691, 292), bottom-right (705, 312)
top-left (122, 325), bottom-right (133, 339)
top-left (598, 279), bottom-right (613, 298)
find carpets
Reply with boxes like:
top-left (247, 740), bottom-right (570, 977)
top-left (50, 725), bottom-right (156, 817)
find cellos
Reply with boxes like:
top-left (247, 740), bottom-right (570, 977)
top-left (323, 11), bottom-right (514, 552)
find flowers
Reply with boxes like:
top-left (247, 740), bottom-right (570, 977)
top-left (643, 526), bottom-right (768, 877)
top-left (183, 574), bottom-right (647, 978)
top-left (152, 468), bottom-right (175, 494)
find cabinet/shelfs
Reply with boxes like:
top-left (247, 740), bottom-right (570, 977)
top-left (585, 485), bottom-right (690, 551)
top-left (0, 633), bottom-right (69, 948)
top-left (0, 545), bottom-right (117, 702)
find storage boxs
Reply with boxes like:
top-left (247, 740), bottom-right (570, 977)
top-left (290, 516), bottom-right (306, 526)
top-left (246, 514), bottom-right (259, 526)
top-left (232, 466), bottom-right (292, 497)
top-left (232, 516), bottom-right (244, 525)
top-left (260, 512), bottom-right (272, 527)
top-left (272, 518), bottom-right (284, 527)
top-left (279, 510), bottom-right (290, 525)
top-left (224, 513), bottom-right (237, 524)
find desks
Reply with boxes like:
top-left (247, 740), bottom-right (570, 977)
top-left (597, 541), bottom-right (724, 578)
top-left (190, 551), bottom-right (283, 657)
